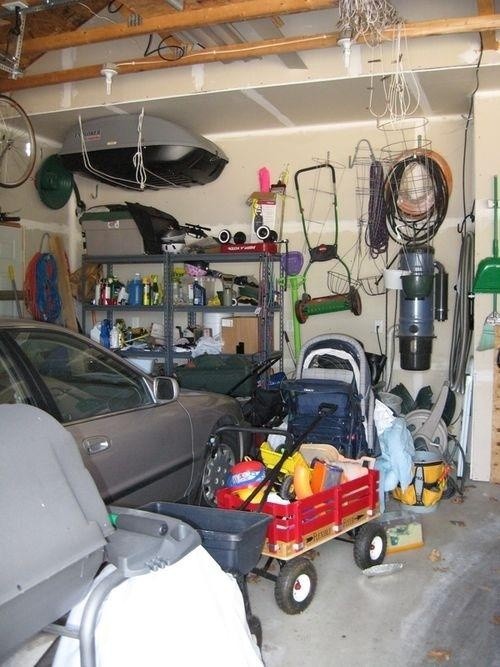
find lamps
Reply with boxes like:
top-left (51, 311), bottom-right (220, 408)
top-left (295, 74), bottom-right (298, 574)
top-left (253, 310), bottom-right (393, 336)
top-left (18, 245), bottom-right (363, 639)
top-left (97, 59), bottom-right (123, 96)
top-left (337, 25), bottom-right (361, 70)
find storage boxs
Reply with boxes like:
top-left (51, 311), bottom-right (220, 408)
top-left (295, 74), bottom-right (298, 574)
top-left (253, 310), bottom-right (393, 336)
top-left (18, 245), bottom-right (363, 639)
top-left (80, 210), bottom-right (169, 256)
top-left (215, 313), bottom-right (275, 354)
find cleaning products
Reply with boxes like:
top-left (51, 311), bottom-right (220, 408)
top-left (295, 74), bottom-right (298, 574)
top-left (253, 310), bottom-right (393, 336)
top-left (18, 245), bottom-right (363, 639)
top-left (94, 273), bottom-right (159, 306)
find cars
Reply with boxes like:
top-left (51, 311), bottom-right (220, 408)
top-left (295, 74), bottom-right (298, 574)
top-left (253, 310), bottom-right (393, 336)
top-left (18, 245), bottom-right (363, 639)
top-left (0, 315), bottom-right (251, 515)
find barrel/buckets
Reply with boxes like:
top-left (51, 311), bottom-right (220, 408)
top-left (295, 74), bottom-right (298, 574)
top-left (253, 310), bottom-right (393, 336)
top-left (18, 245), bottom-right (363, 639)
top-left (90, 319), bottom-right (122, 349)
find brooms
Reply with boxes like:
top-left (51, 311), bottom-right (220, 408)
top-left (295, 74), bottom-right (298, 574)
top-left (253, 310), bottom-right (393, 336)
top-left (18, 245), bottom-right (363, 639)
top-left (476, 175), bottom-right (500, 351)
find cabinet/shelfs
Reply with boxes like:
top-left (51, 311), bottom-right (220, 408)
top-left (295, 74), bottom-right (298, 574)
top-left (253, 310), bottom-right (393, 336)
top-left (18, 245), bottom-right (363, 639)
top-left (73, 246), bottom-right (285, 391)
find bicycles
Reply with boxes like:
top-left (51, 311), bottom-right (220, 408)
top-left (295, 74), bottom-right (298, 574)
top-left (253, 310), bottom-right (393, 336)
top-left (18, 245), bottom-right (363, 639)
top-left (0, 94), bottom-right (37, 192)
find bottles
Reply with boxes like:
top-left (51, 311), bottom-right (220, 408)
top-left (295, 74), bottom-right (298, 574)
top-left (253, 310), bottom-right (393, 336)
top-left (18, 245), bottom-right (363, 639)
top-left (224, 287), bottom-right (232, 305)
top-left (94, 274), bottom-right (121, 306)
top-left (129, 272), bottom-right (159, 306)
top-left (90, 319), bottom-right (133, 347)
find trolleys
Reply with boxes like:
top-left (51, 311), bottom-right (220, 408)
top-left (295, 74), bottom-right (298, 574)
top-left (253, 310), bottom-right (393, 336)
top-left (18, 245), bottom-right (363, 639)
top-left (204, 424), bottom-right (390, 616)
top-left (289, 162), bottom-right (361, 325)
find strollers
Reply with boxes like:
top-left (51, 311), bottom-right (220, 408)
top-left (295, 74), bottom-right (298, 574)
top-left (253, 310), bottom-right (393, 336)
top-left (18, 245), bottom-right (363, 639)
top-left (278, 332), bottom-right (389, 464)
